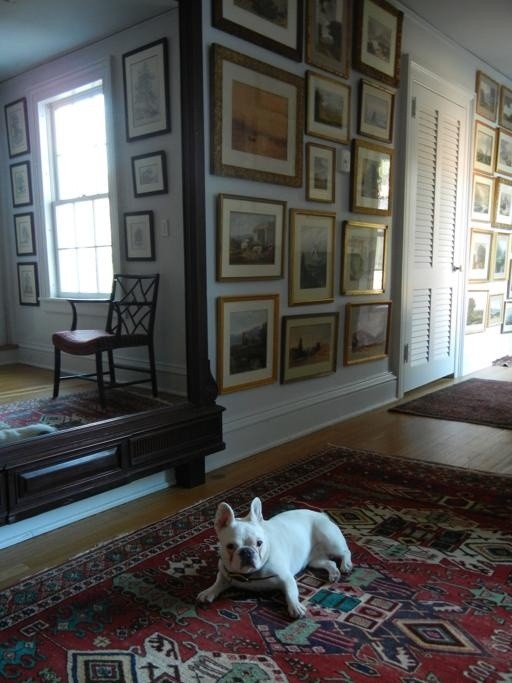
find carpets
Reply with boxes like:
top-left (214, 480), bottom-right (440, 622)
top-left (0, 386), bottom-right (185, 443)
top-left (388, 377), bottom-right (512, 430)
top-left (1, 441), bottom-right (512, 683)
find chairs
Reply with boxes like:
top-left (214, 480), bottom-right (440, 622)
top-left (52, 273), bottom-right (160, 416)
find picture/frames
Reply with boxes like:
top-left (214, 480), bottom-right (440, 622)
top-left (306, 141), bottom-right (337, 205)
top-left (14, 211), bottom-right (36, 256)
top-left (17, 262), bottom-right (39, 306)
top-left (4, 96), bottom-right (30, 159)
top-left (350, 139), bottom-right (394, 216)
top-left (122, 36), bottom-right (171, 143)
top-left (297, 1), bottom-right (403, 87)
top-left (287, 209), bottom-right (337, 306)
top-left (217, 293), bottom-right (279, 394)
top-left (340, 220), bottom-right (389, 296)
top-left (210, 1), bottom-right (303, 63)
top-left (304, 69), bottom-right (351, 144)
top-left (342, 300), bottom-right (392, 366)
top-left (9, 160), bottom-right (33, 207)
top-left (131, 150), bottom-right (168, 198)
top-left (280, 313), bottom-right (339, 384)
top-left (124, 210), bottom-right (155, 262)
top-left (215, 193), bottom-right (288, 283)
top-left (464, 69), bottom-right (512, 336)
top-left (357, 78), bottom-right (396, 144)
top-left (210, 42), bottom-right (303, 188)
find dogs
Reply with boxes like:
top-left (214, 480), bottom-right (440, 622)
top-left (195, 496), bottom-right (355, 620)
